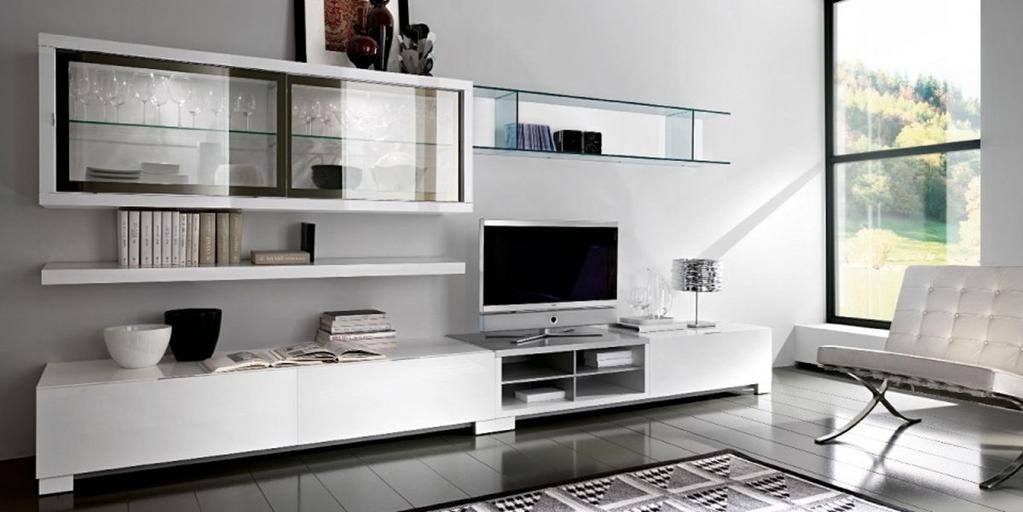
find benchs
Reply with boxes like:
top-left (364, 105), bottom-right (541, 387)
top-left (793, 255), bottom-right (1023, 495)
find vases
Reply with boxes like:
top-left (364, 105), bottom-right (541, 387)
top-left (363, 0), bottom-right (395, 71)
top-left (344, 27), bottom-right (378, 70)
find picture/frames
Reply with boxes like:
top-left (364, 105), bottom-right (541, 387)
top-left (289, 0), bottom-right (415, 78)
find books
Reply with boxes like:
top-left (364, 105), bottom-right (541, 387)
top-left (116, 208), bottom-right (243, 267)
top-left (197, 309), bottom-right (400, 375)
top-left (515, 388), bottom-right (566, 403)
top-left (504, 123), bottom-right (557, 152)
top-left (584, 349), bottom-right (632, 361)
top-left (585, 357), bottom-right (633, 368)
top-left (251, 250), bottom-right (311, 265)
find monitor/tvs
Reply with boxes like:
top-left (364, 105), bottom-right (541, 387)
top-left (477, 218), bottom-right (622, 344)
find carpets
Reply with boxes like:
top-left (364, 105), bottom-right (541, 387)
top-left (378, 436), bottom-right (926, 512)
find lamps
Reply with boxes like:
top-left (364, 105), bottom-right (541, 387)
top-left (676, 255), bottom-right (720, 330)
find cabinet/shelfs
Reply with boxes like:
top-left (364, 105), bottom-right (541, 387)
top-left (22, 314), bottom-right (497, 501)
top-left (24, 27), bottom-right (470, 289)
top-left (464, 82), bottom-right (738, 169)
top-left (440, 315), bottom-right (775, 439)
